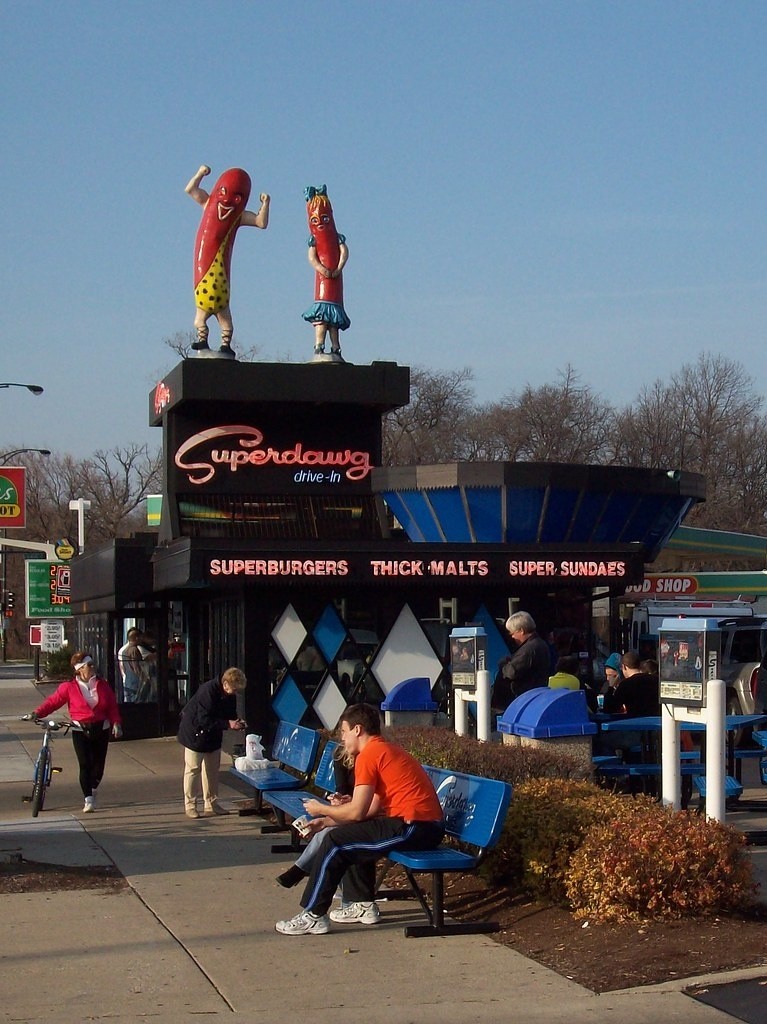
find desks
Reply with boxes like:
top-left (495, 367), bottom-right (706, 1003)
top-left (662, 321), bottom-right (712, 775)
top-left (601, 712), bottom-right (767, 817)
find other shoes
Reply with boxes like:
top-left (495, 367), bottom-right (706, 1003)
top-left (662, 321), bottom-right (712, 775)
top-left (186, 809), bottom-right (200, 818)
top-left (204, 804), bottom-right (229, 815)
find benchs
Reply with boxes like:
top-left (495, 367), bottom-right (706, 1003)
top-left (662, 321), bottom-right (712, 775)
top-left (229, 721), bottom-right (328, 833)
top-left (370, 763), bottom-right (510, 937)
top-left (261, 740), bottom-right (356, 855)
top-left (591, 730), bottom-right (767, 817)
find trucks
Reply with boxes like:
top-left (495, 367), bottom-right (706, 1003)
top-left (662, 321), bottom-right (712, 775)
top-left (626, 598), bottom-right (755, 668)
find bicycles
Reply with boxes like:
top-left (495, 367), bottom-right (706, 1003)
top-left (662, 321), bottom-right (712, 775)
top-left (17, 715), bottom-right (85, 818)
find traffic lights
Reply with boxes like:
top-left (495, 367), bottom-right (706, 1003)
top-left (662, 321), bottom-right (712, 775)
top-left (5, 610), bottom-right (14, 619)
top-left (8, 592), bottom-right (17, 609)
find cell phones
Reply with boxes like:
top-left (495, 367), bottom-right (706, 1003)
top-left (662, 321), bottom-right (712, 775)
top-left (584, 682), bottom-right (592, 689)
top-left (298, 797), bottom-right (312, 803)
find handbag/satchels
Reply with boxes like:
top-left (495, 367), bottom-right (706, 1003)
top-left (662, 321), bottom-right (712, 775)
top-left (246, 734), bottom-right (266, 760)
top-left (235, 757), bottom-right (272, 771)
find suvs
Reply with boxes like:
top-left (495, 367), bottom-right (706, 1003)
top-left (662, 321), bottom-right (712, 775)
top-left (720, 618), bottom-right (767, 749)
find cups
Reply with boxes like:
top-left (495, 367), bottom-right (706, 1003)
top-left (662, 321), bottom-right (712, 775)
top-left (292, 816), bottom-right (311, 837)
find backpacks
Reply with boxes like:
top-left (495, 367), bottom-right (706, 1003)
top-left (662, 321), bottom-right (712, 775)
top-left (490, 664), bottom-right (517, 711)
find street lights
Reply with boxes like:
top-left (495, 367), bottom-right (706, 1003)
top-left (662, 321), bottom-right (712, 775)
top-left (68, 496), bottom-right (93, 556)
top-left (1, 380), bottom-right (52, 661)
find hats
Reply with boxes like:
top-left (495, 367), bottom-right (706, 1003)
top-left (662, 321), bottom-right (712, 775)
top-left (605, 652), bottom-right (625, 681)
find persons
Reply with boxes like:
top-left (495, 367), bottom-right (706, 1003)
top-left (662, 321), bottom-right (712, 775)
top-left (118, 627), bottom-right (170, 726)
top-left (176, 667), bottom-right (244, 818)
top-left (26, 652), bottom-right (123, 813)
top-left (301, 184), bottom-right (351, 354)
top-left (184, 165), bottom-right (271, 356)
top-left (491, 611), bottom-right (695, 788)
top-left (274, 702), bottom-right (445, 935)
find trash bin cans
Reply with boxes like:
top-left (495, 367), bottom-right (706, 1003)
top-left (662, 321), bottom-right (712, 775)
top-left (381, 676), bottom-right (437, 728)
top-left (494, 684), bottom-right (598, 762)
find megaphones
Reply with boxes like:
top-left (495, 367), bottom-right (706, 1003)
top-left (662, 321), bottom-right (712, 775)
top-left (666, 469), bottom-right (680, 482)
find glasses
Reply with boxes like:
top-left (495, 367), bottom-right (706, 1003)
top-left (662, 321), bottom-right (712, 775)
top-left (82, 661), bottom-right (93, 667)
top-left (508, 629), bottom-right (519, 636)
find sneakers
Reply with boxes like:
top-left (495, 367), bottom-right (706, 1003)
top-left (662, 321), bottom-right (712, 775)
top-left (329, 902), bottom-right (382, 925)
top-left (91, 788), bottom-right (97, 808)
top-left (275, 910), bottom-right (331, 935)
top-left (83, 796), bottom-right (95, 813)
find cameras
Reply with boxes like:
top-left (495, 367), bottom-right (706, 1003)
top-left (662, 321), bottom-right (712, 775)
top-left (330, 792), bottom-right (342, 801)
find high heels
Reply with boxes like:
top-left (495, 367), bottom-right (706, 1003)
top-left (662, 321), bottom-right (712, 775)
top-left (274, 864), bottom-right (305, 888)
